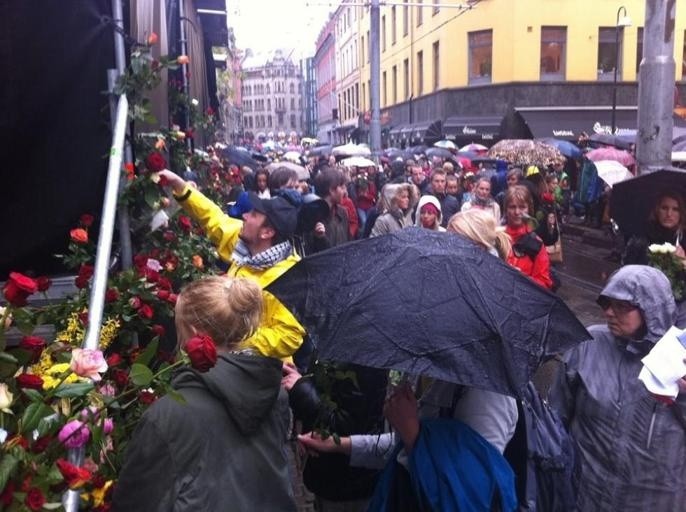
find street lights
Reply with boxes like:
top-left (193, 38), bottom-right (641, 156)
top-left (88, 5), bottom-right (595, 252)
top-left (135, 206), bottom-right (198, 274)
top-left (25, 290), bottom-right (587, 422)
top-left (611, 5), bottom-right (634, 136)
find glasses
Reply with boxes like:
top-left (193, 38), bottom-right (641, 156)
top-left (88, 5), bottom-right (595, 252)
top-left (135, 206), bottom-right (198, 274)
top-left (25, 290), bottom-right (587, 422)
top-left (599, 298), bottom-right (641, 315)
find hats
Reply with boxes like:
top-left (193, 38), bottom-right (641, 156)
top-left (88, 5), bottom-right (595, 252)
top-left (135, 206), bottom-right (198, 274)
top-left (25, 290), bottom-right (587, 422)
top-left (526, 164), bottom-right (540, 178)
top-left (248, 190), bottom-right (297, 236)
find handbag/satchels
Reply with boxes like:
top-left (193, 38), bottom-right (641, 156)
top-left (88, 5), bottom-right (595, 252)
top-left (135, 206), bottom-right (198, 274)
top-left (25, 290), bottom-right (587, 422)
top-left (546, 238), bottom-right (564, 262)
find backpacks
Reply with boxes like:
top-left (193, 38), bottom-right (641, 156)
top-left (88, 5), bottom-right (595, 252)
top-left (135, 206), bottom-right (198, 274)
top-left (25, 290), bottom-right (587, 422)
top-left (527, 379), bottom-right (579, 512)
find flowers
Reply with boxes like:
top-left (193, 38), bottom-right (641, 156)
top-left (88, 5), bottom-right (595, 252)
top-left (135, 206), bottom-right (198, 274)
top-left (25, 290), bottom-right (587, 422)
top-left (99, 31), bottom-right (217, 231)
top-left (645, 239), bottom-right (684, 301)
top-left (539, 189), bottom-right (555, 214)
top-left (0, 226), bottom-right (216, 512)
